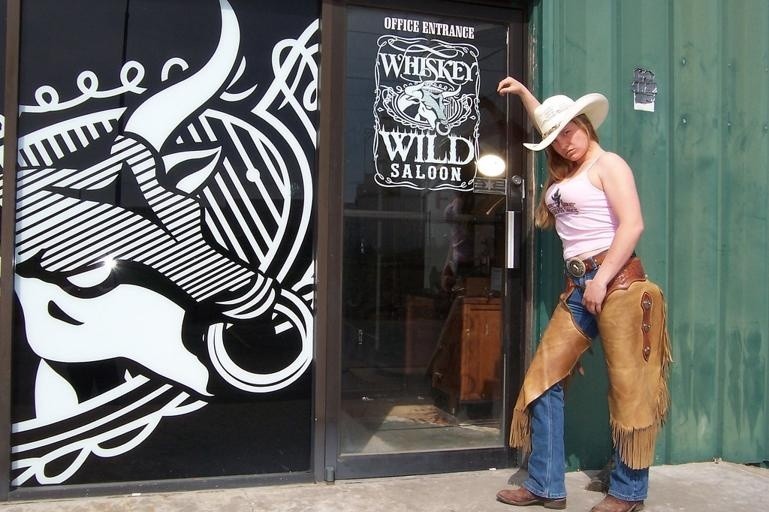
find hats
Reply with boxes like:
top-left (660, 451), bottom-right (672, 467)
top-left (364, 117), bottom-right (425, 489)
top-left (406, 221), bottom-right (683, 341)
top-left (522, 93), bottom-right (610, 152)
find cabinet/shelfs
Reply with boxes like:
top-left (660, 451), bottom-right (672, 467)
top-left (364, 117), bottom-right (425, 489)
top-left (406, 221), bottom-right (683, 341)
top-left (431, 296), bottom-right (504, 424)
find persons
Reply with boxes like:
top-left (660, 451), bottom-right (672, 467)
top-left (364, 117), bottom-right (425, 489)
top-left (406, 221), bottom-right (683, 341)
top-left (443, 170), bottom-right (489, 285)
top-left (497, 76), bottom-right (670, 511)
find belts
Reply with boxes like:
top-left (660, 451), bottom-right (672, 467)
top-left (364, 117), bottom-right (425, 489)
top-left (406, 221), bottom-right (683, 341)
top-left (567, 250), bottom-right (608, 278)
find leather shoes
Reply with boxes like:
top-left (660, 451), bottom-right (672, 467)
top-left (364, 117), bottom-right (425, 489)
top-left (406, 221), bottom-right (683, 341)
top-left (497, 485), bottom-right (566, 510)
top-left (590, 494), bottom-right (645, 512)
